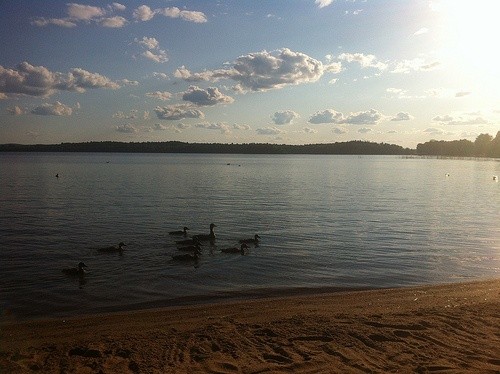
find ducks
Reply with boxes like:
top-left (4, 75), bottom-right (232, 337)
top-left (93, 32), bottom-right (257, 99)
top-left (192, 223), bottom-right (217, 240)
top-left (239, 234), bottom-right (261, 243)
top-left (220, 243), bottom-right (251, 253)
top-left (174, 239), bottom-right (202, 261)
top-left (167, 226), bottom-right (191, 235)
top-left (98, 241), bottom-right (127, 252)
top-left (78, 262), bottom-right (91, 275)
